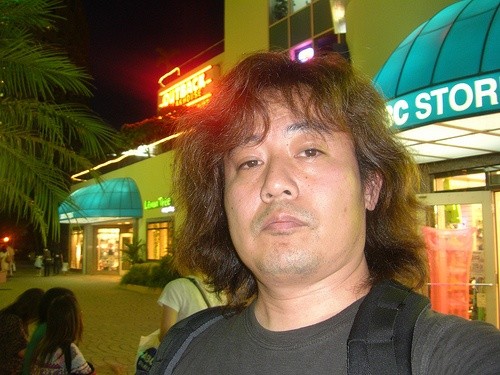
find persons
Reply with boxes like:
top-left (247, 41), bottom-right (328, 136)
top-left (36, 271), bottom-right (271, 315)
top-left (0, 287), bottom-right (44, 375)
top-left (22, 287), bottom-right (75, 375)
top-left (145, 49), bottom-right (500, 375)
top-left (53, 249), bottom-right (63, 274)
top-left (62, 257), bottom-right (69, 275)
top-left (156, 243), bottom-right (233, 344)
top-left (0, 242), bottom-right (17, 278)
top-left (31, 292), bottom-right (95, 375)
top-left (35, 252), bottom-right (44, 276)
top-left (43, 247), bottom-right (51, 276)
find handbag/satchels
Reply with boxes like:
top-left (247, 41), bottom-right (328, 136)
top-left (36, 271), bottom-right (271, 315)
top-left (134, 329), bottom-right (160, 375)
